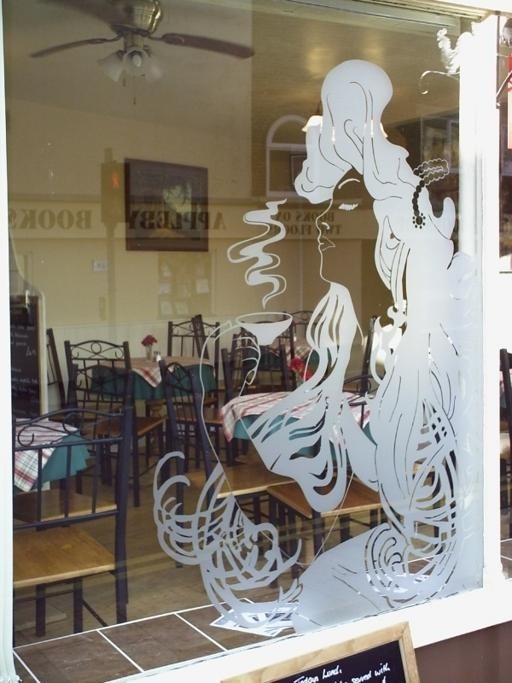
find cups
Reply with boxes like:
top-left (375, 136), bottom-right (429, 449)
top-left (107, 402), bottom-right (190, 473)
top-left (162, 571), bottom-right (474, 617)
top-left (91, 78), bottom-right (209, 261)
top-left (235, 311), bottom-right (294, 346)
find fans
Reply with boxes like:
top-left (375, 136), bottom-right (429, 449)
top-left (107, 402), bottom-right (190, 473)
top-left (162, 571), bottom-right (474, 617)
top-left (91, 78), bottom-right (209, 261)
top-left (29, 0), bottom-right (255, 60)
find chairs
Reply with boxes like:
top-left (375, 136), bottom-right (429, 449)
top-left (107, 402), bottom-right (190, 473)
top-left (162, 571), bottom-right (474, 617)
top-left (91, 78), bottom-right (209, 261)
top-left (12, 310), bottom-right (456, 639)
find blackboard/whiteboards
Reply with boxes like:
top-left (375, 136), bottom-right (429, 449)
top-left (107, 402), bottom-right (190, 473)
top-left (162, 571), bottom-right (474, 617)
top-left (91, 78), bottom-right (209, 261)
top-left (12, 292), bottom-right (48, 420)
top-left (208, 622), bottom-right (418, 683)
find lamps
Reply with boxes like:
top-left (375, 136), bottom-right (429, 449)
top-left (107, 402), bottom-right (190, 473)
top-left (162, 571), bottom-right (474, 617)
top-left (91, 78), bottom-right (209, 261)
top-left (95, 47), bottom-right (168, 82)
